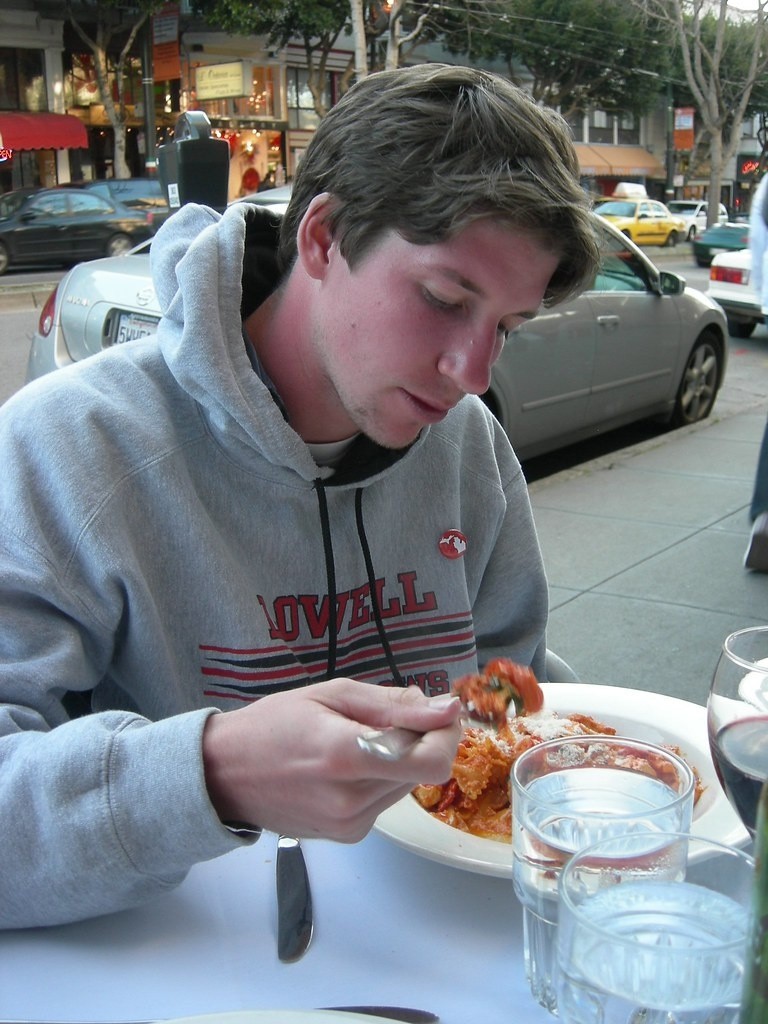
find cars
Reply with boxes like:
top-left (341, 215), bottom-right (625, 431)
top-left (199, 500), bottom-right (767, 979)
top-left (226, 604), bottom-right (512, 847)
top-left (689, 223), bottom-right (752, 269)
top-left (704, 248), bottom-right (768, 341)
top-left (54, 178), bottom-right (169, 234)
top-left (0, 188), bottom-right (156, 276)
top-left (594, 182), bottom-right (686, 248)
top-left (664, 200), bottom-right (729, 243)
top-left (22, 180), bottom-right (729, 470)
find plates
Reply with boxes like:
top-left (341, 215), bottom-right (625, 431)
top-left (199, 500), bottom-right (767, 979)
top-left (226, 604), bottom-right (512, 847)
top-left (373, 679), bottom-right (762, 885)
top-left (149, 1009), bottom-right (415, 1024)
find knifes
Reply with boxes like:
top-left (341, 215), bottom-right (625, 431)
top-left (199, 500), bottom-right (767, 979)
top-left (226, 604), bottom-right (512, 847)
top-left (277, 832), bottom-right (314, 965)
top-left (309, 1006), bottom-right (441, 1024)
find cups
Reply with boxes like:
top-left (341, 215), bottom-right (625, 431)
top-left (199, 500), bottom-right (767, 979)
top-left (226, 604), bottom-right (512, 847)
top-left (707, 625), bottom-right (768, 851)
top-left (509, 735), bottom-right (696, 1017)
top-left (552, 831), bottom-right (757, 1024)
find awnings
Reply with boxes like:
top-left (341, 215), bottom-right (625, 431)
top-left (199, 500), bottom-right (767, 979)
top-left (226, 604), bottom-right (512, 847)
top-left (0, 111), bottom-right (89, 153)
top-left (575, 146), bottom-right (657, 178)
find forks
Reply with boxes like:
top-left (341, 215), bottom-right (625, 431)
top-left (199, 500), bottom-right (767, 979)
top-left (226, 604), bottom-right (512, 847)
top-left (355, 677), bottom-right (519, 760)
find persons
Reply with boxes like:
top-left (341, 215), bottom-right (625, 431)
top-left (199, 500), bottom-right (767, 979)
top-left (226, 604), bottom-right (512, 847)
top-left (743, 171), bottom-right (768, 574)
top-left (0, 64), bottom-right (598, 930)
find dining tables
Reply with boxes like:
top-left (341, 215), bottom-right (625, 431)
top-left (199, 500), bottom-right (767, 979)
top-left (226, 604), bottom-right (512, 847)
top-left (0, 825), bottom-right (767, 1023)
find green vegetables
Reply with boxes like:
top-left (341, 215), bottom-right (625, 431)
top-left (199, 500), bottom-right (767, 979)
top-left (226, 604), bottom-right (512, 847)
top-left (486, 675), bottom-right (523, 718)
